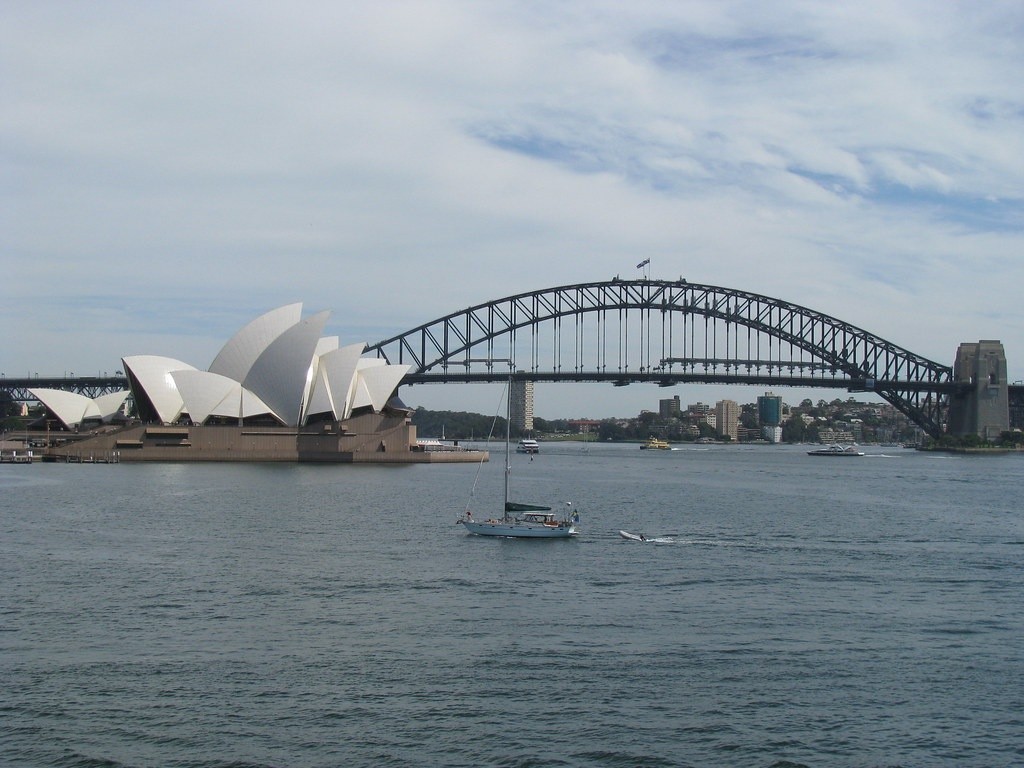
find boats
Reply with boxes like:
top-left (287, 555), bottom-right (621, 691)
top-left (515, 434), bottom-right (540, 454)
top-left (806, 444), bottom-right (865, 456)
top-left (638, 436), bottom-right (672, 451)
top-left (618, 529), bottom-right (650, 542)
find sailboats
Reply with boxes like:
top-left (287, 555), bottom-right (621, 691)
top-left (453, 376), bottom-right (584, 538)
